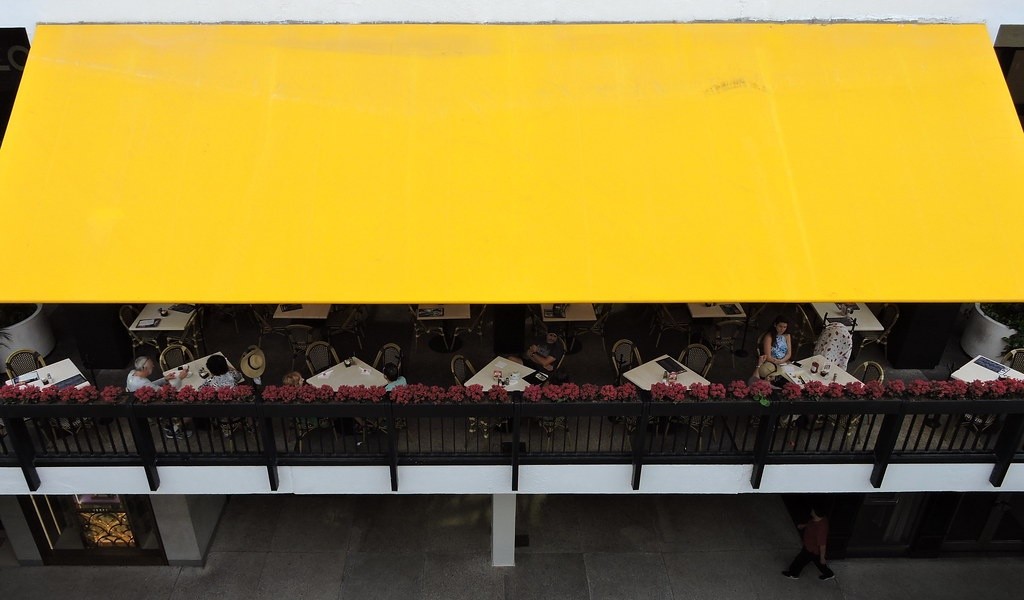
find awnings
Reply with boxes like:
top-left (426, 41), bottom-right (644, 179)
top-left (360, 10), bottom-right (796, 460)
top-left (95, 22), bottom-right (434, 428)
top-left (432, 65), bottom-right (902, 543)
top-left (1, 20), bottom-right (1024, 303)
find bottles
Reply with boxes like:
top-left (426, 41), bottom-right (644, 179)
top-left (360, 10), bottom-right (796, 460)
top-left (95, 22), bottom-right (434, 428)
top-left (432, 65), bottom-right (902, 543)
top-left (505, 378), bottom-right (510, 385)
top-left (663, 371), bottom-right (668, 380)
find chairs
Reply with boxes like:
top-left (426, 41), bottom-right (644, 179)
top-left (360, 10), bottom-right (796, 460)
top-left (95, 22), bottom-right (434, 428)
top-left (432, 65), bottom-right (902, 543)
top-left (855, 304), bottom-right (899, 362)
top-left (119, 305), bottom-right (162, 361)
top-left (1001, 348), bottom-right (1024, 374)
top-left (738, 303), bottom-right (769, 337)
top-left (373, 342), bottom-right (403, 379)
top-left (650, 304), bottom-right (695, 348)
top-left (699, 318), bottom-right (744, 369)
top-left (527, 304), bottom-right (565, 349)
top-left (522, 335), bottom-right (567, 374)
top-left (159, 345), bottom-right (194, 372)
top-left (570, 303), bottom-right (614, 354)
top-left (305, 341), bottom-right (340, 377)
top-left (250, 304), bottom-right (290, 349)
top-left (787, 304), bottom-right (821, 360)
top-left (167, 307), bottom-right (208, 359)
top-left (327, 304), bottom-right (368, 354)
top-left (449, 303), bottom-right (489, 352)
top-left (678, 344), bottom-right (713, 377)
top-left (612, 339), bottom-right (643, 386)
top-left (851, 360), bottom-right (884, 387)
top-left (450, 354), bottom-right (476, 387)
top-left (5, 348), bottom-right (46, 380)
top-left (284, 325), bottom-right (321, 371)
top-left (408, 305), bottom-right (450, 353)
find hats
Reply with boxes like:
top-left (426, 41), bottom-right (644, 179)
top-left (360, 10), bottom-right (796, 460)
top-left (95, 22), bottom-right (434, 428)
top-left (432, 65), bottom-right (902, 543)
top-left (758, 362), bottom-right (783, 378)
top-left (240, 348), bottom-right (266, 378)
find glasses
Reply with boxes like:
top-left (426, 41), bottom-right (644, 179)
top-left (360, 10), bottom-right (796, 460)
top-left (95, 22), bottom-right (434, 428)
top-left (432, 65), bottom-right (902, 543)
top-left (143, 355), bottom-right (151, 367)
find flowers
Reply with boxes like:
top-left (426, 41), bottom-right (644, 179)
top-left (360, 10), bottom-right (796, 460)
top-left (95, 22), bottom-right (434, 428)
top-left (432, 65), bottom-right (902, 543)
top-left (0, 376), bottom-right (1024, 406)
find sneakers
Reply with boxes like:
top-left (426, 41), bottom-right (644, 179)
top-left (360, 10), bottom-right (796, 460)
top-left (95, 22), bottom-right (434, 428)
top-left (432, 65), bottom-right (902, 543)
top-left (166, 429), bottom-right (194, 440)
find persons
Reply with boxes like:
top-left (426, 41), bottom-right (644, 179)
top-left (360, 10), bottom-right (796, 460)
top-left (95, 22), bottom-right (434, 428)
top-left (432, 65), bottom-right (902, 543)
top-left (763, 315), bottom-right (792, 382)
top-left (527, 329), bottom-right (562, 371)
top-left (125, 356), bottom-right (192, 440)
top-left (237, 344), bottom-right (266, 385)
top-left (749, 306), bottom-right (761, 321)
top-left (196, 355), bottom-right (243, 438)
top-left (781, 504), bottom-right (836, 581)
top-left (747, 355), bottom-right (783, 389)
top-left (281, 371), bottom-right (305, 387)
top-left (383, 362), bottom-right (407, 392)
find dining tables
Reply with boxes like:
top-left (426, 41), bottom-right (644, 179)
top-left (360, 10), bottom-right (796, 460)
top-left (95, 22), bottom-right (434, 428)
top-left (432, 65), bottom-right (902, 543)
top-left (464, 356), bottom-right (548, 392)
top-left (272, 303), bottom-right (331, 320)
top-left (163, 352), bottom-right (244, 392)
top-left (416, 304), bottom-right (471, 320)
top-left (624, 355), bottom-right (711, 390)
top-left (686, 303), bottom-right (747, 317)
top-left (129, 303), bottom-right (208, 331)
top-left (951, 355), bottom-right (1024, 385)
top-left (781, 355), bottom-right (865, 388)
top-left (540, 304), bottom-right (597, 320)
top-left (5, 358), bottom-right (91, 393)
top-left (306, 356), bottom-right (388, 392)
top-left (810, 303), bottom-right (884, 330)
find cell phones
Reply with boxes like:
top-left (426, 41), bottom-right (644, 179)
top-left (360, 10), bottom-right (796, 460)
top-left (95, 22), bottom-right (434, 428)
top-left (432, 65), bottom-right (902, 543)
top-left (791, 361), bottom-right (804, 367)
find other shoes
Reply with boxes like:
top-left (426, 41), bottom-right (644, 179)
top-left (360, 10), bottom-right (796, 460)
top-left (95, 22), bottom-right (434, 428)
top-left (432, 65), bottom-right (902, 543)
top-left (819, 574), bottom-right (836, 581)
top-left (782, 570), bottom-right (800, 580)
top-left (806, 420), bottom-right (825, 431)
